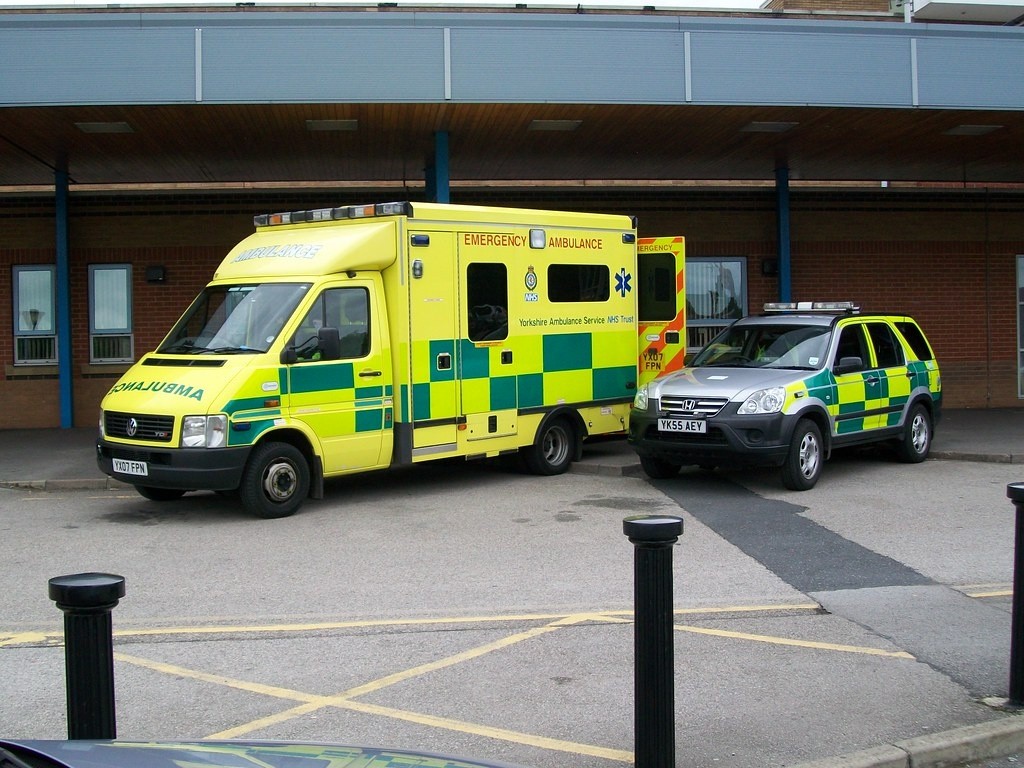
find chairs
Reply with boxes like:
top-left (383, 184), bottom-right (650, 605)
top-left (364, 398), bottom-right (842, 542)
top-left (257, 295), bottom-right (299, 352)
top-left (338, 296), bottom-right (367, 357)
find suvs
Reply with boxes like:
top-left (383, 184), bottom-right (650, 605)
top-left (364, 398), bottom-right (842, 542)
top-left (626, 302), bottom-right (944, 492)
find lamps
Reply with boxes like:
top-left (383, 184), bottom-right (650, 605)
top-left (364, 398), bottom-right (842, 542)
top-left (762, 256), bottom-right (781, 275)
top-left (146, 263), bottom-right (167, 282)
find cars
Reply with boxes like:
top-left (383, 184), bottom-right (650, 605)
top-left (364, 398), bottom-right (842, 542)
top-left (1, 739), bottom-right (513, 768)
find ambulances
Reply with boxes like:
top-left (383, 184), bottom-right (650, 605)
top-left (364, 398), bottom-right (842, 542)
top-left (94, 201), bottom-right (689, 519)
